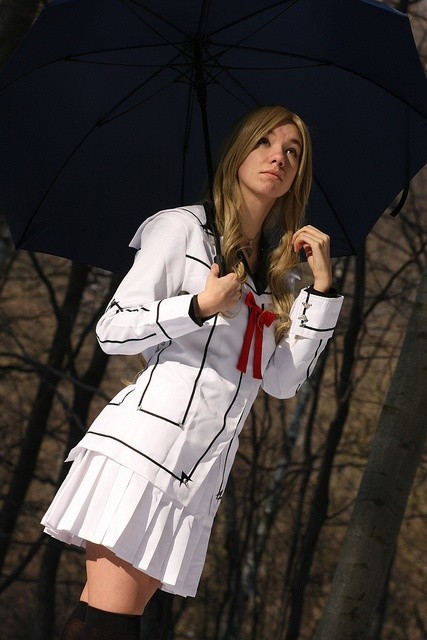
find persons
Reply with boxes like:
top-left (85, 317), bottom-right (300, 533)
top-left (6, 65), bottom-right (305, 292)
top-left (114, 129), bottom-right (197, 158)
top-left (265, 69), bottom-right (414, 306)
top-left (39, 106), bottom-right (345, 639)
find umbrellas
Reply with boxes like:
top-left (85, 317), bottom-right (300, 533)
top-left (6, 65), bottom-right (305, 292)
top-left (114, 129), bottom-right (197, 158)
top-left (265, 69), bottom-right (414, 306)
top-left (0, 1), bottom-right (427, 320)
